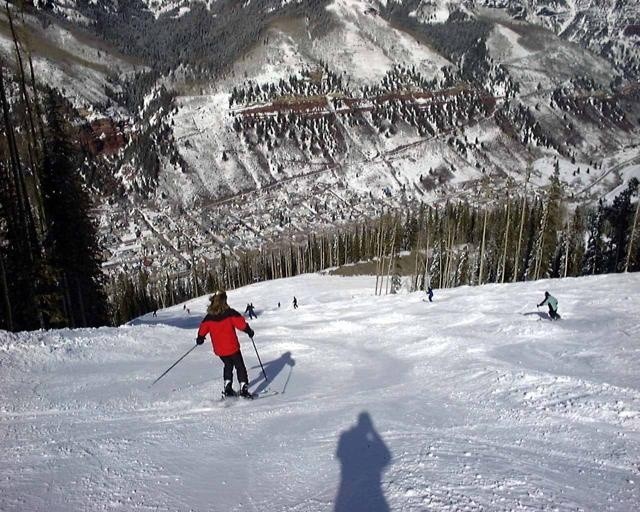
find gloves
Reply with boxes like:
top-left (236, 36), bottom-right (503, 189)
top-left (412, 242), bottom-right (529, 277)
top-left (248, 329), bottom-right (254, 337)
top-left (196, 336), bottom-right (204, 344)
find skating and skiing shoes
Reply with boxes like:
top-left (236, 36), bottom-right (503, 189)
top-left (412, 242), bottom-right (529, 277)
top-left (237, 382), bottom-right (253, 400)
top-left (221, 379), bottom-right (237, 399)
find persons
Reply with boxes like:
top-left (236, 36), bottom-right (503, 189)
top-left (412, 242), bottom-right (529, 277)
top-left (426, 286), bottom-right (433, 302)
top-left (245, 303), bottom-right (257, 320)
top-left (293, 297), bottom-right (298, 309)
top-left (537, 292), bottom-right (560, 320)
top-left (197, 291), bottom-right (255, 400)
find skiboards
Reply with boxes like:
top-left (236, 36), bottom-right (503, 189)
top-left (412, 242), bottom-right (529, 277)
top-left (204, 388), bottom-right (279, 408)
